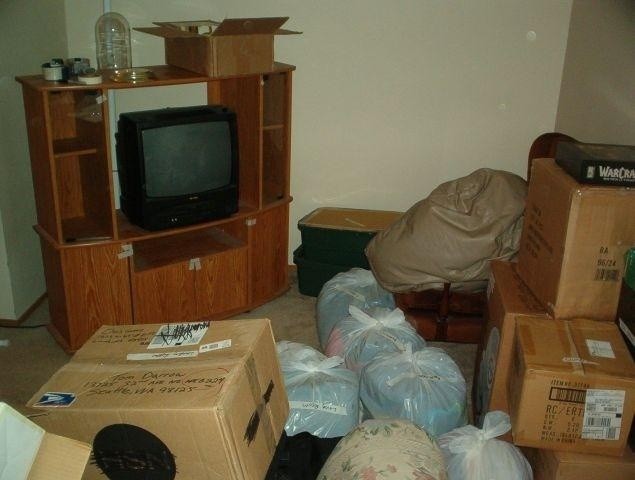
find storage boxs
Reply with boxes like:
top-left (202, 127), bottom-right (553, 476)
top-left (24, 318), bottom-right (291, 480)
top-left (131, 16), bottom-right (304, 78)
top-left (1, 401), bottom-right (94, 480)
top-left (297, 206), bottom-right (407, 270)
top-left (294, 244), bottom-right (349, 297)
top-left (470, 144), bottom-right (635, 480)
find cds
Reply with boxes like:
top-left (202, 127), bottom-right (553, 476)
top-left (110, 66), bottom-right (156, 83)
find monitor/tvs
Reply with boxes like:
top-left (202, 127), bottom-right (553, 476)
top-left (114, 103), bottom-right (242, 233)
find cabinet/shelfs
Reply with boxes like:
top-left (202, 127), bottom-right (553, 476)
top-left (15, 60), bottom-right (296, 355)
